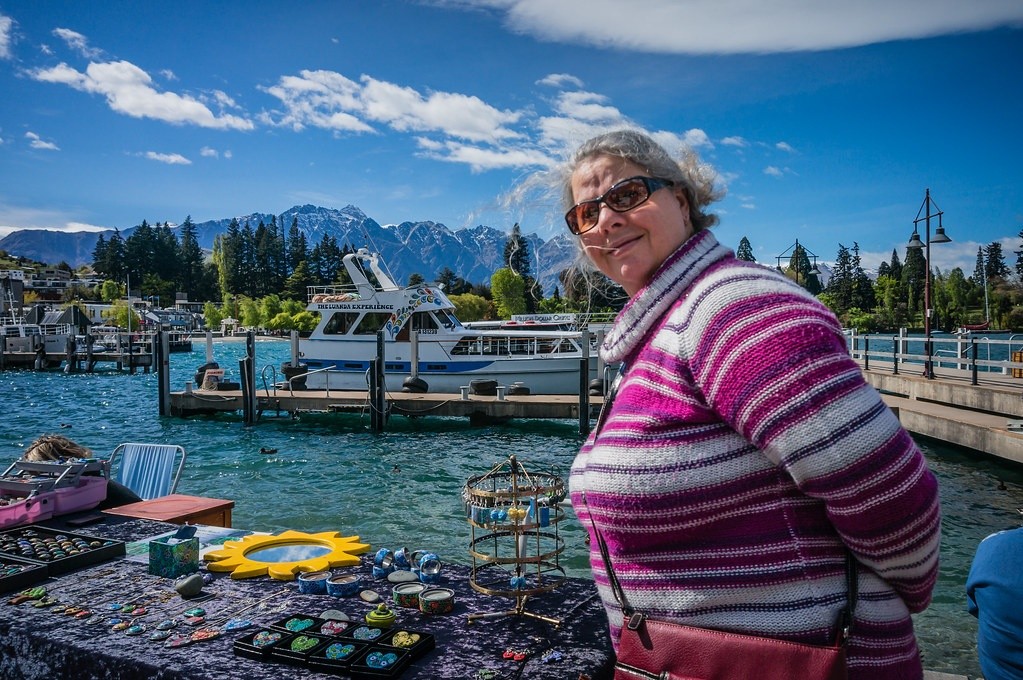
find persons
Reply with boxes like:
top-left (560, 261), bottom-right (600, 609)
top-left (966, 526), bottom-right (1023, 680)
top-left (24, 436), bottom-right (143, 509)
top-left (564, 131), bottom-right (941, 680)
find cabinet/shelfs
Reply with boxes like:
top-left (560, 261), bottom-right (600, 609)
top-left (102, 494), bottom-right (235, 529)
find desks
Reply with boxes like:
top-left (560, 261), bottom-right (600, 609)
top-left (0, 520), bottom-right (627, 680)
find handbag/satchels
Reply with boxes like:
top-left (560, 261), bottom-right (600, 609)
top-left (612, 616), bottom-right (847, 680)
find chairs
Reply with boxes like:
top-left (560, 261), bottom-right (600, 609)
top-left (108, 443), bottom-right (187, 500)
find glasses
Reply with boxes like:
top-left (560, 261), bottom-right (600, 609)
top-left (565, 176), bottom-right (674, 235)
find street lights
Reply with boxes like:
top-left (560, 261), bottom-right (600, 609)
top-left (775, 256), bottom-right (822, 285)
top-left (906, 212), bottom-right (953, 379)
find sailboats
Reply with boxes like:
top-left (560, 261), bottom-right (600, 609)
top-left (122, 274), bottom-right (152, 366)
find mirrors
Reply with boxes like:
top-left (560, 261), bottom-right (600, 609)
top-left (204, 530), bottom-right (370, 580)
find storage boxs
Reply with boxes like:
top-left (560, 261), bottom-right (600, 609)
top-left (149, 533), bottom-right (199, 579)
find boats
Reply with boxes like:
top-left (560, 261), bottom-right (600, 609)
top-left (842, 328), bottom-right (851, 335)
top-left (299, 242), bottom-right (621, 394)
top-left (955, 328), bottom-right (971, 336)
top-left (1, 286), bottom-right (108, 352)
top-left (931, 329), bottom-right (943, 334)
